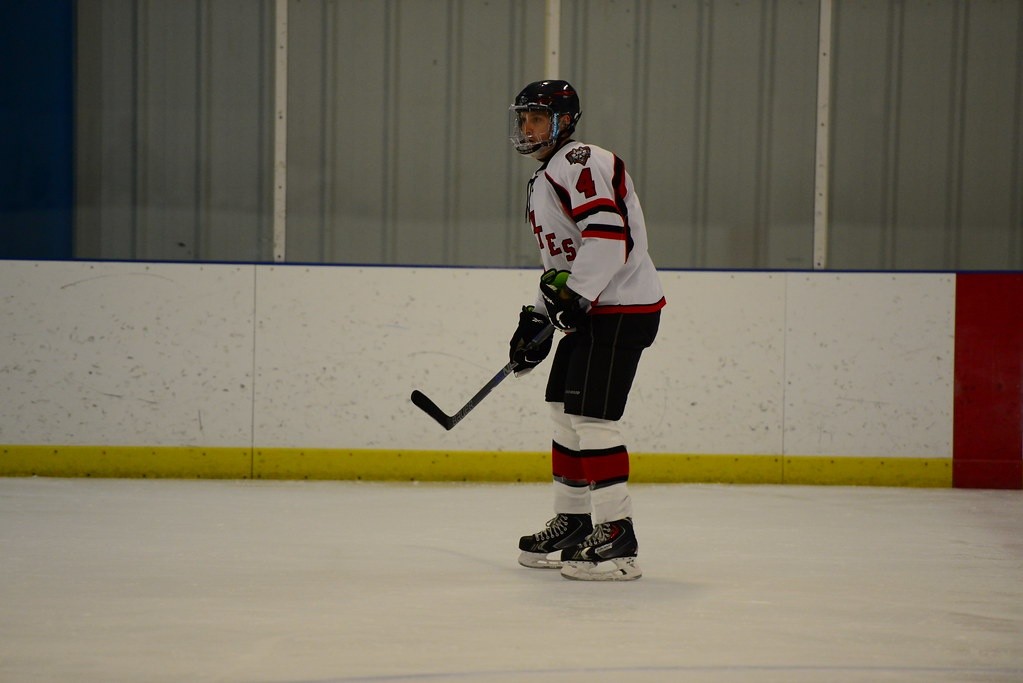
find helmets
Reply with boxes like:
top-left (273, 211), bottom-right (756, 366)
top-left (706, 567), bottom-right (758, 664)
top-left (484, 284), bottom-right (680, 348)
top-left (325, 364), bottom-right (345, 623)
top-left (507, 80), bottom-right (581, 158)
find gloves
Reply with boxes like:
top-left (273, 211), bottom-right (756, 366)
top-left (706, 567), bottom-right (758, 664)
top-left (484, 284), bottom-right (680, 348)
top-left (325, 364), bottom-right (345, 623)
top-left (540, 268), bottom-right (593, 333)
top-left (508, 305), bottom-right (556, 379)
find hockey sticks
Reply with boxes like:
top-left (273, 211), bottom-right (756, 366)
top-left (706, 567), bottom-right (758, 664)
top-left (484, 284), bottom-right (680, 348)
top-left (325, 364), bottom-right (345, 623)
top-left (411, 321), bottom-right (555, 430)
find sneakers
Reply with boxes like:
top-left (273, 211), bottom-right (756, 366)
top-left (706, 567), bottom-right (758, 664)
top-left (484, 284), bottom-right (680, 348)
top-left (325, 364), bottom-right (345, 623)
top-left (560, 517), bottom-right (643, 582)
top-left (517, 514), bottom-right (595, 569)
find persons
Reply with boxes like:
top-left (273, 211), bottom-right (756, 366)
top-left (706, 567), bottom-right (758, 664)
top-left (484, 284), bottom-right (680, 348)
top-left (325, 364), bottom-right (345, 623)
top-left (508, 78), bottom-right (668, 580)
